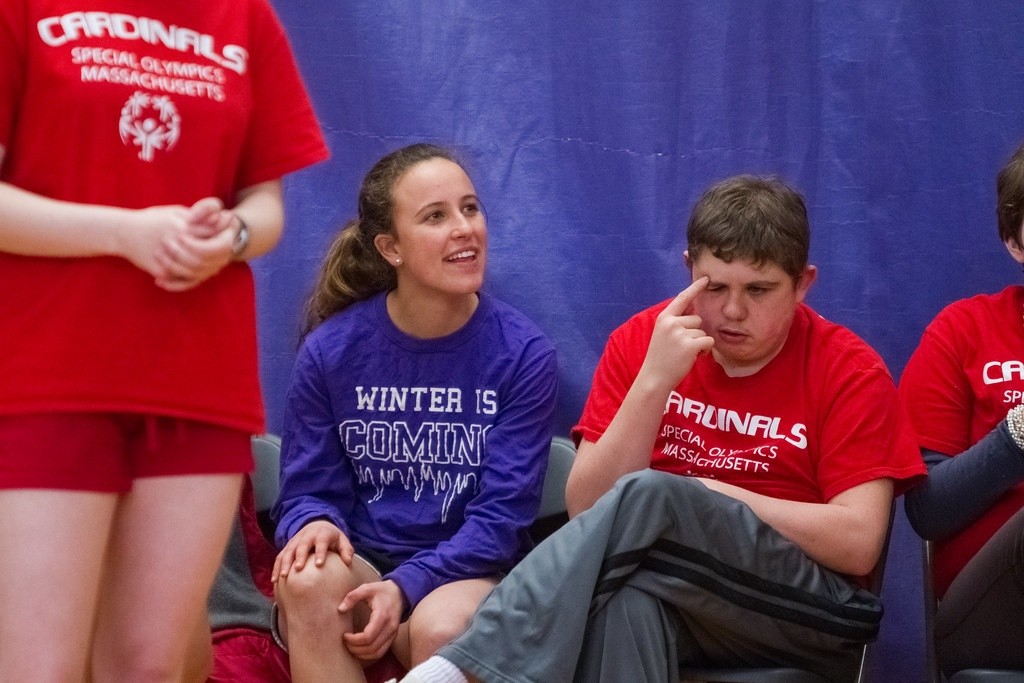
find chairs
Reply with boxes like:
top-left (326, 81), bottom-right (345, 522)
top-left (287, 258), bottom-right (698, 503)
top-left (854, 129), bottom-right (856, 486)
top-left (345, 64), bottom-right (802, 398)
top-left (657, 486), bottom-right (900, 683)
top-left (918, 522), bottom-right (1024, 683)
top-left (238, 424), bottom-right (579, 524)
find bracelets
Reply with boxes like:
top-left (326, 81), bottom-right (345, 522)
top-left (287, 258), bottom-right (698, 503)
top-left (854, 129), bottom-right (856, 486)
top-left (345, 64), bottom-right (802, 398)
top-left (232, 217), bottom-right (249, 256)
top-left (1006, 403), bottom-right (1024, 450)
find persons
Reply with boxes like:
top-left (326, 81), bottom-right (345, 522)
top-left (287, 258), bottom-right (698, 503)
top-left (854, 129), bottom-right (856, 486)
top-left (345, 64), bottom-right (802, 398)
top-left (0, 0), bottom-right (333, 683)
top-left (275, 143), bottom-right (562, 683)
top-left (894, 147), bottom-right (1024, 683)
top-left (397, 173), bottom-right (928, 683)
